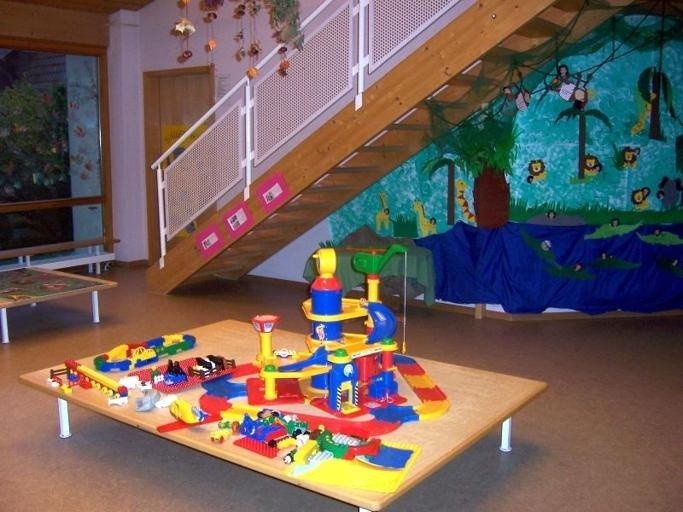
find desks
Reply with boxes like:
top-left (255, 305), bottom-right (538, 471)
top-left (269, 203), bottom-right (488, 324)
top-left (302, 244), bottom-right (435, 310)
top-left (0, 267), bottom-right (117, 343)
top-left (18, 319), bottom-right (549, 512)
